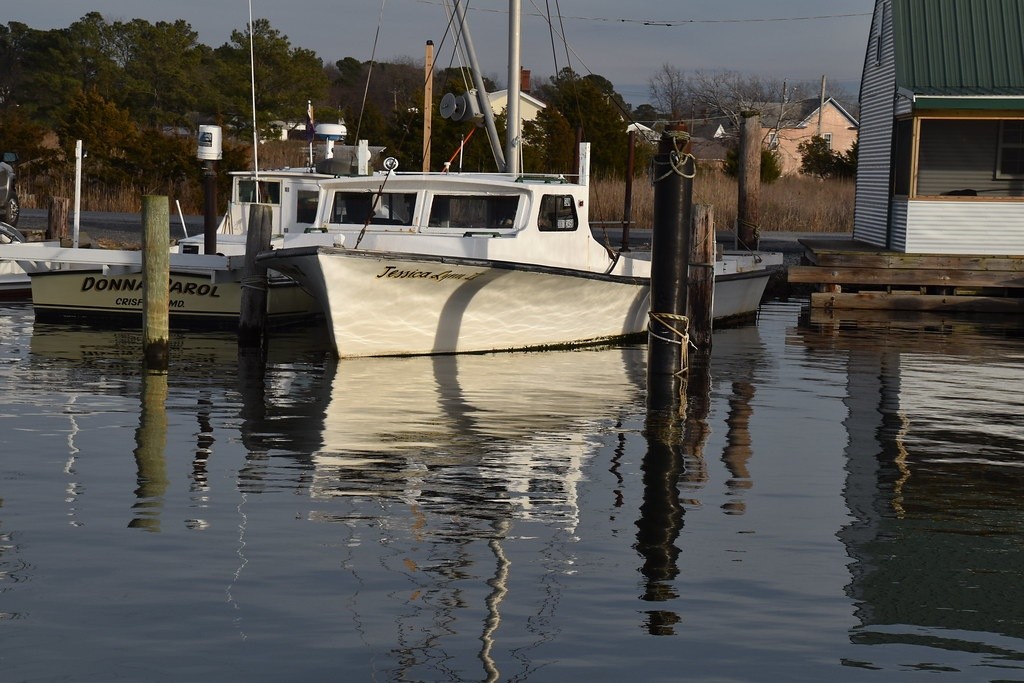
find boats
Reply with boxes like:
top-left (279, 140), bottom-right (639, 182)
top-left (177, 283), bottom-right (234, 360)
top-left (0, 222), bottom-right (60, 300)
top-left (253, 0), bottom-right (786, 359)
top-left (0, 0), bottom-right (404, 332)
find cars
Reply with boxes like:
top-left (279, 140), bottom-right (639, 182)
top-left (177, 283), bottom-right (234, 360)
top-left (0, 162), bottom-right (20, 228)
